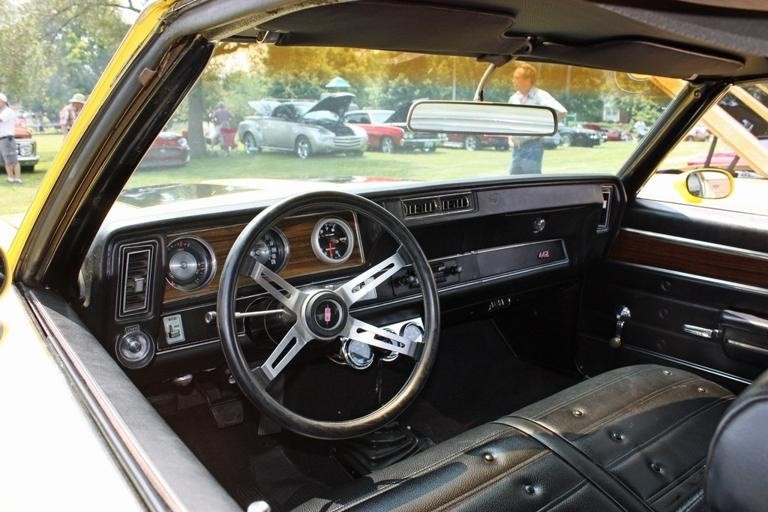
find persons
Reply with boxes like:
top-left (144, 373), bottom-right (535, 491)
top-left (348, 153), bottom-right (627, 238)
top-left (0, 93), bottom-right (22, 183)
top-left (18, 112), bottom-right (50, 133)
top-left (508, 64), bottom-right (568, 175)
top-left (212, 102), bottom-right (235, 155)
top-left (59, 94), bottom-right (87, 137)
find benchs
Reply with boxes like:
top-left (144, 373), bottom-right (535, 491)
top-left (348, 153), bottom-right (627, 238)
top-left (289, 363), bottom-right (768, 511)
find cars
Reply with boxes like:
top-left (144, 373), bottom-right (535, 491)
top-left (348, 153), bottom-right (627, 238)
top-left (138, 126), bottom-right (190, 169)
top-left (688, 147), bottom-right (768, 177)
top-left (237, 91), bottom-right (510, 158)
top-left (638, 126), bottom-right (712, 142)
top-left (0, 123), bottom-right (39, 173)
top-left (544, 122), bottom-right (632, 150)
